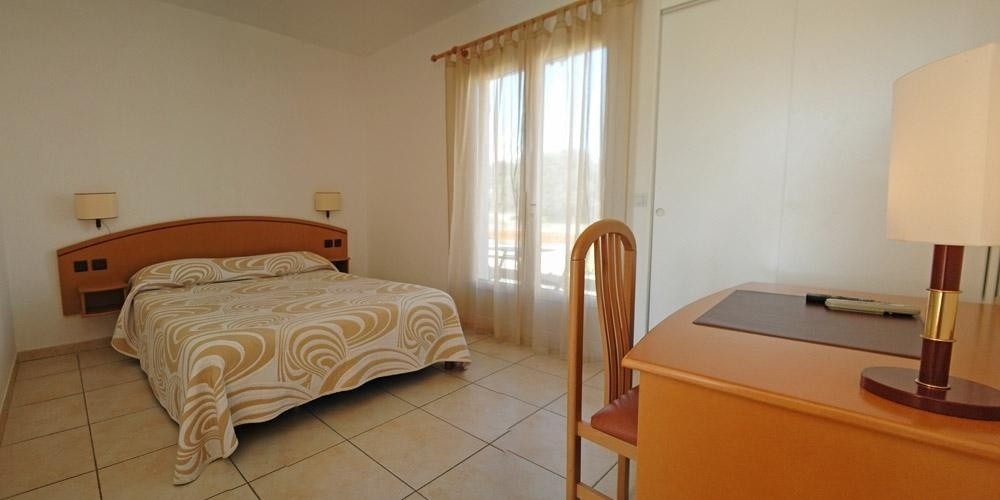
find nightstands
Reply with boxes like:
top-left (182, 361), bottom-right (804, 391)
top-left (77, 280), bottom-right (130, 318)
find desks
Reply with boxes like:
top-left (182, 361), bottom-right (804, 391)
top-left (618, 280), bottom-right (997, 497)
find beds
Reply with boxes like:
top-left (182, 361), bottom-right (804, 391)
top-left (56, 214), bottom-right (472, 488)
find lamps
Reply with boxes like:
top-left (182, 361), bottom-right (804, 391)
top-left (853, 44), bottom-right (999, 424)
top-left (312, 190), bottom-right (345, 219)
top-left (72, 191), bottom-right (120, 229)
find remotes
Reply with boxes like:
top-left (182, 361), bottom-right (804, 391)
top-left (825, 299), bottom-right (922, 316)
top-left (806, 292), bottom-right (880, 306)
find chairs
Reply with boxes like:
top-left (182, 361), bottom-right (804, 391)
top-left (561, 216), bottom-right (643, 500)
